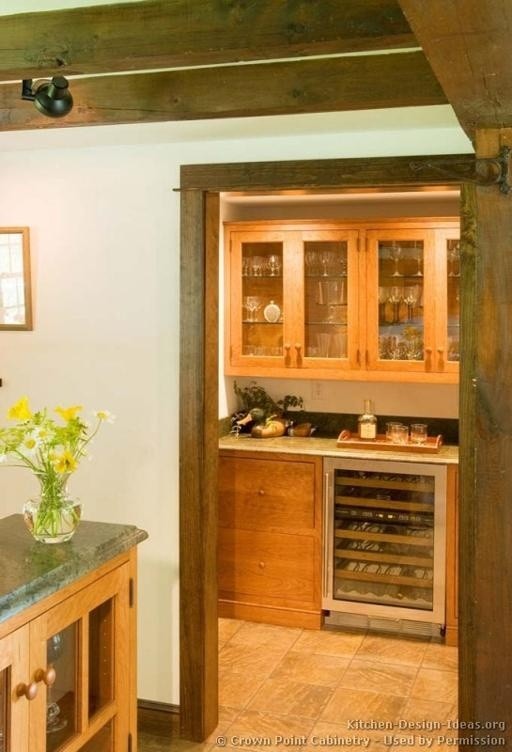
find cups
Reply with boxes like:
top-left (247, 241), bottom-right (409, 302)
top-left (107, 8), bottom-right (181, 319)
top-left (380, 334), bottom-right (423, 360)
top-left (243, 344), bottom-right (283, 356)
top-left (385, 422), bottom-right (428, 445)
top-left (316, 332), bottom-right (346, 358)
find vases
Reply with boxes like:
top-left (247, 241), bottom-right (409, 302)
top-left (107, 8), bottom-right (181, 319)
top-left (21, 473), bottom-right (81, 543)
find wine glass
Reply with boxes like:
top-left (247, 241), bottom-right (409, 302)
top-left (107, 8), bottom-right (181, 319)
top-left (312, 281), bottom-right (347, 324)
top-left (243, 297), bottom-right (261, 322)
top-left (306, 251), bottom-right (347, 276)
top-left (379, 242), bottom-right (423, 276)
top-left (242, 253), bottom-right (281, 278)
top-left (378, 285), bottom-right (422, 326)
top-left (447, 240), bottom-right (461, 278)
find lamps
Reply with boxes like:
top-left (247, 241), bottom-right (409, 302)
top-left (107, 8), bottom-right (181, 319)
top-left (21, 75), bottom-right (74, 117)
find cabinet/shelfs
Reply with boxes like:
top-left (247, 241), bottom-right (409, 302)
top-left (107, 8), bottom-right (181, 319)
top-left (223, 215), bottom-right (460, 382)
top-left (0, 544), bottom-right (138, 752)
top-left (216, 450), bottom-right (324, 631)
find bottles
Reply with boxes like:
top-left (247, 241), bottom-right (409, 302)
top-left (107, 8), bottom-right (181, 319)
top-left (263, 300), bottom-right (281, 323)
top-left (357, 399), bottom-right (378, 441)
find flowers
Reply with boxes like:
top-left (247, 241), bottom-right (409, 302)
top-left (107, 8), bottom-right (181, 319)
top-left (0, 395), bottom-right (113, 540)
